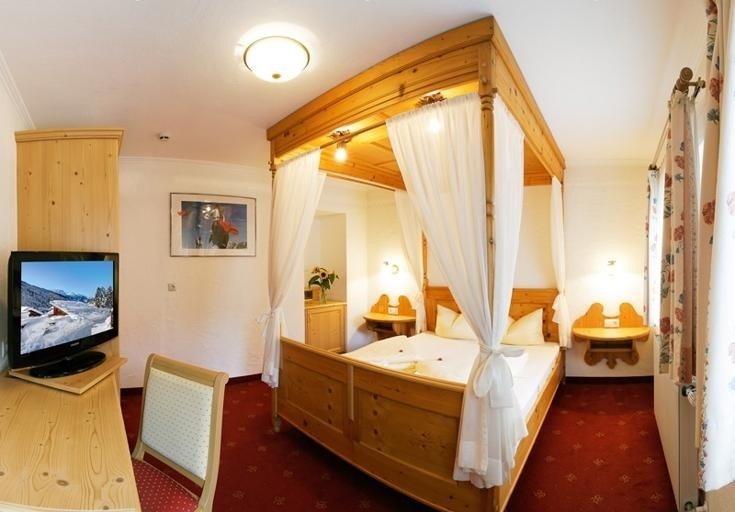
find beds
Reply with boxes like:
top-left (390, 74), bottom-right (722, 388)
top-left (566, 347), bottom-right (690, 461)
top-left (266, 17), bottom-right (565, 512)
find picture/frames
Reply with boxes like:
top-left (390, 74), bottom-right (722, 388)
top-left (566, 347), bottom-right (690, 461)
top-left (170, 192), bottom-right (256, 258)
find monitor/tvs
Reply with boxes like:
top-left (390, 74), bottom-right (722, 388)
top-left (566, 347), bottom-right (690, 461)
top-left (7, 249), bottom-right (120, 378)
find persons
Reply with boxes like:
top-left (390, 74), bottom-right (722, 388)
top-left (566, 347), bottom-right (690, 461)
top-left (206, 205), bottom-right (241, 249)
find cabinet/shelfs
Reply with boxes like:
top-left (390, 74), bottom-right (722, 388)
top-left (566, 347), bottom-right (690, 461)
top-left (14, 126), bottom-right (125, 357)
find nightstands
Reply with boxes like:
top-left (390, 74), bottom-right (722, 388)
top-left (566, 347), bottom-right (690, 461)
top-left (363, 295), bottom-right (416, 338)
top-left (573, 304), bottom-right (651, 368)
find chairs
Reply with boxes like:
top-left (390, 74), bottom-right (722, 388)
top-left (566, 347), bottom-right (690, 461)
top-left (131, 354), bottom-right (229, 512)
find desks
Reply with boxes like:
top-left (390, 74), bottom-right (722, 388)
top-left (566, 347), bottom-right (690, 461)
top-left (303, 300), bottom-right (345, 353)
top-left (0, 357), bottom-right (141, 512)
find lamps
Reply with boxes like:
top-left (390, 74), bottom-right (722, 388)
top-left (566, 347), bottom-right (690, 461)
top-left (336, 139), bottom-right (346, 163)
top-left (235, 23), bottom-right (320, 84)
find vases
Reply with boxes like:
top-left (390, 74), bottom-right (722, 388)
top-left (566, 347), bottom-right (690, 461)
top-left (319, 289), bottom-right (326, 304)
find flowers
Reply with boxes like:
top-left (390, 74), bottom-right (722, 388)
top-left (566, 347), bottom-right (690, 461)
top-left (309, 266), bottom-right (339, 290)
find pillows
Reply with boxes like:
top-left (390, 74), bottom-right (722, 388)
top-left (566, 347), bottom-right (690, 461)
top-left (436, 305), bottom-right (547, 346)
